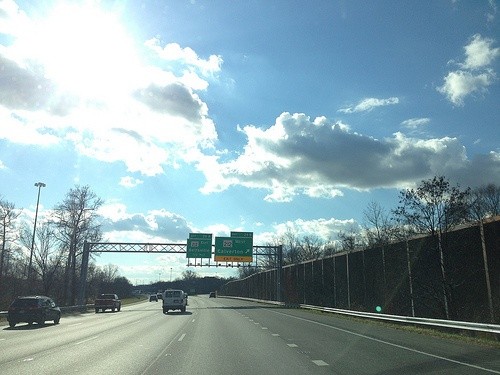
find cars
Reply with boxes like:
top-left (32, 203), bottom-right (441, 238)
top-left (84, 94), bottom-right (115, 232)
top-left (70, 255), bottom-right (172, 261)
top-left (7, 294), bottom-right (61, 328)
top-left (184, 292), bottom-right (187, 306)
top-left (157, 293), bottom-right (162, 299)
top-left (150, 295), bottom-right (158, 302)
top-left (94, 294), bottom-right (121, 313)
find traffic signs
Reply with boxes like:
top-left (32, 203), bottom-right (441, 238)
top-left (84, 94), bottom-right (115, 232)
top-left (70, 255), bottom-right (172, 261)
top-left (186, 233), bottom-right (212, 258)
top-left (214, 232), bottom-right (254, 262)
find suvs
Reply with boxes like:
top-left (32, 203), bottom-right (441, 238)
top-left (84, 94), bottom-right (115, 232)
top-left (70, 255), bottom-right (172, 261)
top-left (209, 292), bottom-right (216, 298)
top-left (162, 289), bottom-right (186, 314)
